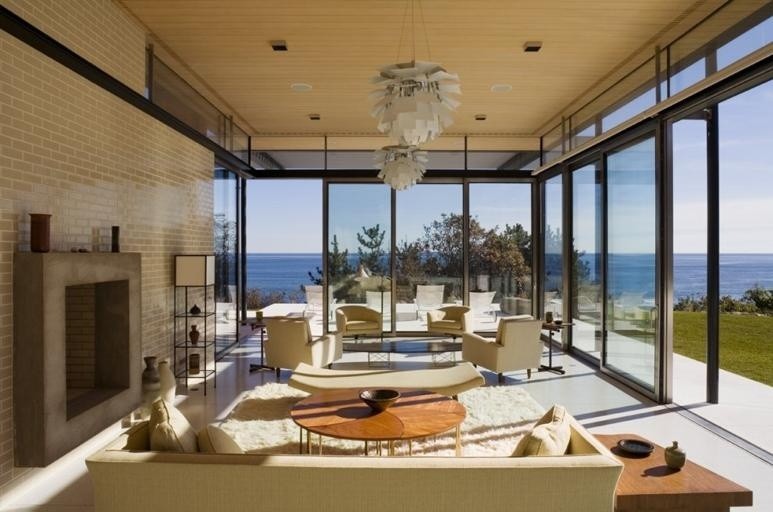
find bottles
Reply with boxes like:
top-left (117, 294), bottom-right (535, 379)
top-left (664, 441), bottom-right (687, 471)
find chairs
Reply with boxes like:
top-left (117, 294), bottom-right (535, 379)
top-left (262, 283), bottom-right (543, 383)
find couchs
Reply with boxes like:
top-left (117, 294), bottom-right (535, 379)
top-left (84, 411), bottom-right (625, 512)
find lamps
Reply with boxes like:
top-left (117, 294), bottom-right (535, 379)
top-left (366, 0), bottom-right (462, 192)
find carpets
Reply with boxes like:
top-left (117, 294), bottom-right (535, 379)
top-left (218, 380), bottom-right (547, 457)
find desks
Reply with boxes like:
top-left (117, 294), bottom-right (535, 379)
top-left (240, 317), bottom-right (274, 372)
top-left (593, 432), bottom-right (752, 512)
top-left (537, 322), bottom-right (575, 374)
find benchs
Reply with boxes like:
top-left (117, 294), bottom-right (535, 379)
top-left (287, 361), bottom-right (486, 402)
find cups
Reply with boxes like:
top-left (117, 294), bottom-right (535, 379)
top-left (546, 311), bottom-right (553, 323)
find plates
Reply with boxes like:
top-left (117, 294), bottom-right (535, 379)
top-left (617, 438), bottom-right (655, 456)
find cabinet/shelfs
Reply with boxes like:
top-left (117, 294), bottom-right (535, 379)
top-left (172, 255), bottom-right (216, 396)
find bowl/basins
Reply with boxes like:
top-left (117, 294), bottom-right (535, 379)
top-left (359, 389), bottom-right (402, 410)
top-left (554, 320), bottom-right (563, 325)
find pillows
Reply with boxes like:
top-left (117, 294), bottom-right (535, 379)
top-left (146, 399), bottom-right (242, 454)
top-left (510, 404), bottom-right (570, 456)
